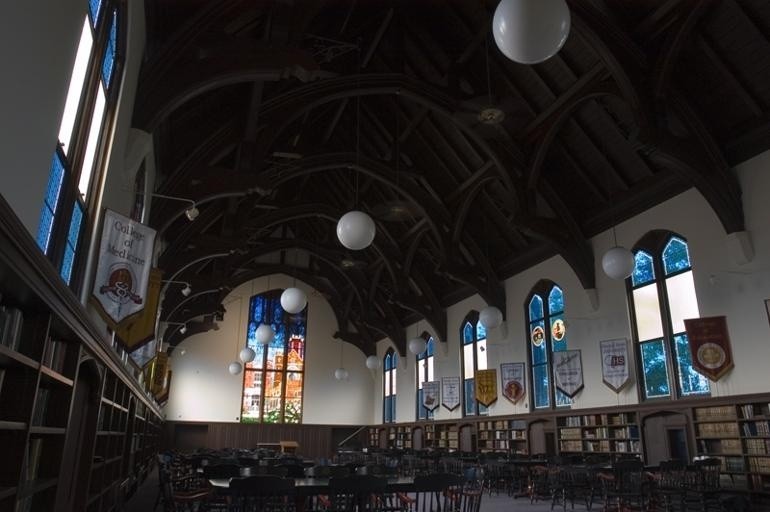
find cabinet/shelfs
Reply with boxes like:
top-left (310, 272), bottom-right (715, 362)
top-left (367, 392), bottom-right (770, 512)
top-left (90, 317), bottom-right (167, 509)
top-left (4, 194), bottom-right (90, 509)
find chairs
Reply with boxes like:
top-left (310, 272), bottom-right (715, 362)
top-left (155, 445), bottom-right (386, 512)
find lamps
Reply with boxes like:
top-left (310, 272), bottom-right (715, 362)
top-left (494, 0), bottom-right (572, 65)
top-left (123, 191), bottom-right (199, 223)
top-left (228, 177), bottom-right (309, 376)
top-left (589, 95), bottom-right (637, 280)
top-left (335, 34), bottom-right (377, 250)
